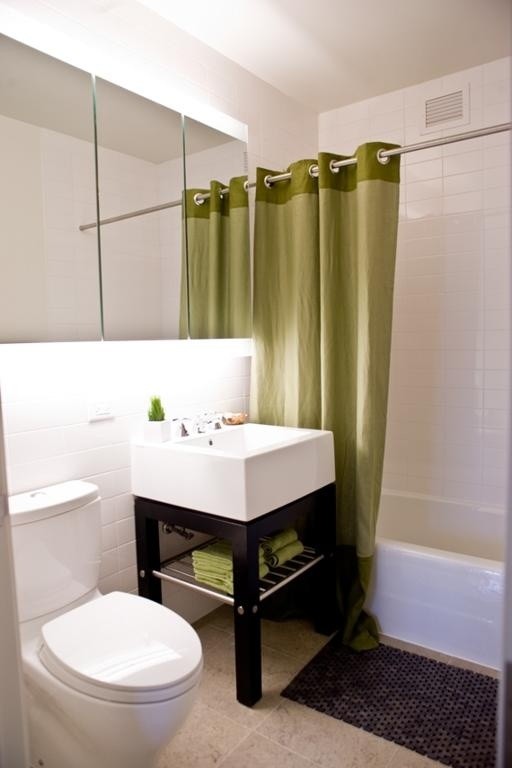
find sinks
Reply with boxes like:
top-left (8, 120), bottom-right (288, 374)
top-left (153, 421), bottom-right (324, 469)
top-left (131, 423), bottom-right (336, 519)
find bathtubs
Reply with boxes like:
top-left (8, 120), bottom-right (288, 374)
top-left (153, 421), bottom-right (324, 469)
top-left (361, 486), bottom-right (507, 673)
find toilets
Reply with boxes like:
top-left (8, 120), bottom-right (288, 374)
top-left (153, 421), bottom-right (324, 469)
top-left (8, 480), bottom-right (203, 767)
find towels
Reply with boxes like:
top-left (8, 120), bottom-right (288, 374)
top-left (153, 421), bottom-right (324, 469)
top-left (193, 525), bottom-right (304, 595)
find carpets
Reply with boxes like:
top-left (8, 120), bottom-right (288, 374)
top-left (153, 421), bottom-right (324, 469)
top-left (279, 630), bottom-right (499, 768)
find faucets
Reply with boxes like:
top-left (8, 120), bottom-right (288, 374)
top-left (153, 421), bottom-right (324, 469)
top-left (197, 411), bottom-right (234, 435)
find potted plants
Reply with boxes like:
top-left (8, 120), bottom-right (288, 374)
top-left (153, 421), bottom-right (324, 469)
top-left (143, 396), bottom-right (170, 442)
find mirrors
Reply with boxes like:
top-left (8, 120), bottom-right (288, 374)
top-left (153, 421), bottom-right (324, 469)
top-left (0, 33), bottom-right (253, 345)
